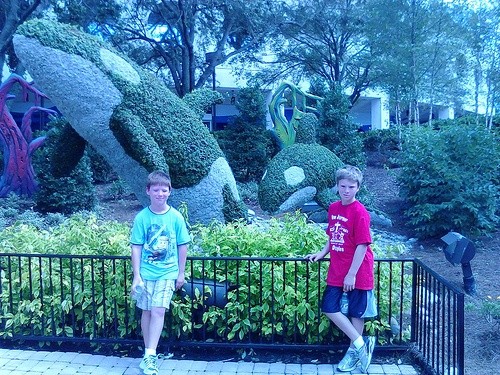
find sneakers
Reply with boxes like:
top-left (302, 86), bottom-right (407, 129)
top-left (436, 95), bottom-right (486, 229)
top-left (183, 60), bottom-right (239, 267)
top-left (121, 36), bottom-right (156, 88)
top-left (337, 347), bottom-right (360, 372)
top-left (139, 354), bottom-right (159, 375)
top-left (357, 336), bottom-right (375, 373)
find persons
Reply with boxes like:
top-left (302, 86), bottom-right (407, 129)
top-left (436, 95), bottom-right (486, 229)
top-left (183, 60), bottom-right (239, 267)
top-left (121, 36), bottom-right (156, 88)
top-left (307, 166), bottom-right (377, 373)
top-left (130, 170), bottom-right (191, 375)
